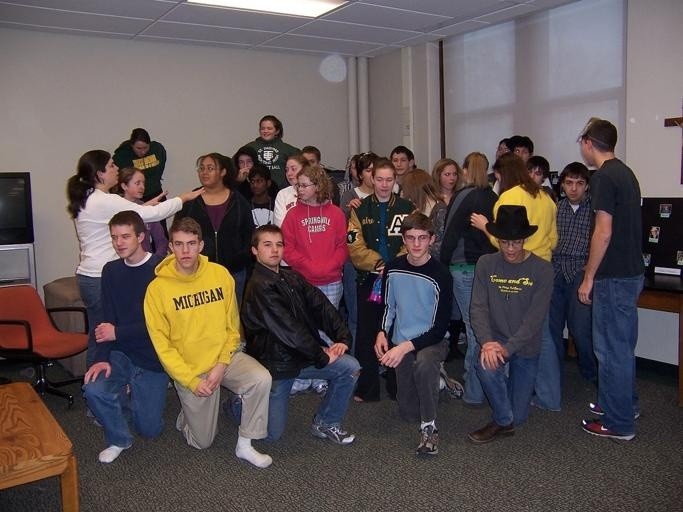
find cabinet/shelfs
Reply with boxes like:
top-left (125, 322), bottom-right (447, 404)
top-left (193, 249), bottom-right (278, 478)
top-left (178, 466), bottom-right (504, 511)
top-left (567, 288), bottom-right (682, 406)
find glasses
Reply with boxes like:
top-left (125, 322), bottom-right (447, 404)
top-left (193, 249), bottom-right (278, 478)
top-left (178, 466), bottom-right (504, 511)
top-left (496, 147), bottom-right (510, 153)
top-left (294, 183), bottom-right (314, 189)
top-left (497, 238), bottom-right (524, 248)
top-left (513, 149), bottom-right (529, 156)
top-left (197, 166), bottom-right (219, 172)
top-left (404, 234), bottom-right (430, 242)
top-left (249, 179), bottom-right (264, 183)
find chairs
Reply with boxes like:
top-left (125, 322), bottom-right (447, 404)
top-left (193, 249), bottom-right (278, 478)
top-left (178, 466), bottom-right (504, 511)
top-left (0, 285), bottom-right (90, 409)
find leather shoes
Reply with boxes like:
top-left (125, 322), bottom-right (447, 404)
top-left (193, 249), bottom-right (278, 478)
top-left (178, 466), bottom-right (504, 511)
top-left (469, 421), bottom-right (515, 443)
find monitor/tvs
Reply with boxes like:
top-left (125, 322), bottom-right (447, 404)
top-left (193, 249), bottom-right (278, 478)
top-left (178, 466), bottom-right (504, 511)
top-left (0, 172), bottom-right (34, 244)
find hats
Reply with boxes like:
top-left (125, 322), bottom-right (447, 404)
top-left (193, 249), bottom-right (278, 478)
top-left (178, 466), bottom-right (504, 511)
top-left (485, 205), bottom-right (539, 240)
top-left (576, 117), bottom-right (617, 149)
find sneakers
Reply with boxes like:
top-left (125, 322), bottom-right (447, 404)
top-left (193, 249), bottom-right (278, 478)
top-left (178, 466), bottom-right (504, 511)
top-left (289, 384), bottom-right (312, 399)
top-left (179, 408), bottom-right (187, 430)
top-left (312, 382), bottom-right (330, 398)
top-left (416, 419), bottom-right (439, 457)
top-left (440, 368), bottom-right (465, 401)
top-left (311, 416), bottom-right (356, 445)
top-left (579, 418), bottom-right (636, 440)
top-left (220, 392), bottom-right (242, 415)
top-left (586, 401), bottom-right (639, 420)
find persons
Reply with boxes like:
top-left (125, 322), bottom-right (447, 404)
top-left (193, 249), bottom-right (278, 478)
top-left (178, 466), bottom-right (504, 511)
top-left (650, 226), bottom-right (659, 239)
top-left (172, 114), bottom-right (563, 411)
top-left (372, 213), bottom-right (466, 459)
top-left (552, 161), bottom-right (596, 392)
top-left (219, 223), bottom-right (361, 448)
top-left (79, 208), bottom-right (169, 465)
top-left (65, 149), bottom-right (206, 428)
top-left (466, 203), bottom-right (556, 447)
top-left (661, 205), bottom-right (670, 213)
top-left (140, 216), bottom-right (274, 473)
top-left (576, 116), bottom-right (646, 441)
top-left (112, 129), bottom-right (170, 259)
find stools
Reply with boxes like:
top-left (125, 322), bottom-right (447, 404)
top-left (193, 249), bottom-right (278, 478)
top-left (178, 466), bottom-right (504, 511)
top-left (42, 276), bottom-right (92, 378)
top-left (0, 381), bottom-right (78, 511)
